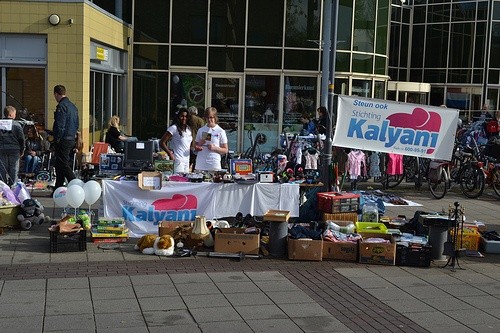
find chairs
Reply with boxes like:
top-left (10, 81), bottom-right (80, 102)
top-left (41, 141), bottom-right (109, 173)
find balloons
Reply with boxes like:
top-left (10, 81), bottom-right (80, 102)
top-left (83, 180), bottom-right (102, 205)
top-left (66, 184), bottom-right (85, 208)
top-left (52, 187), bottom-right (68, 209)
top-left (66, 177), bottom-right (84, 188)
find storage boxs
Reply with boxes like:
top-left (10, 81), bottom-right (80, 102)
top-left (287, 192), bottom-right (429, 267)
top-left (214, 227), bottom-right (261, 254)
top-left (0, 205), bottom-right (20, 227)
top-left (100, 153), bottom-right (124, 171)
top-left (50, 220), bottom-right (87, 253)
top-left (255, 169), bottom-right (273, 183)
top-left (138, 171), bottom-right (163, 190)
top-left (263, 209), bottom-right (290, 221)
top-left (451, 224), bottom-right (500, 254)
top-left (158, 220), bottom-right (195, 239)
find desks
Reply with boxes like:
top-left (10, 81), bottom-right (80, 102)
top-left (101, 180), bottom-right (323, 236)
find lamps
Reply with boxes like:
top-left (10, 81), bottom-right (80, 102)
top-left (127, 36), bottom-right (132, 44)
top-left (49, 13), bottom-right (61, 25)
top-left (191, 215), bottom-right (211, 256)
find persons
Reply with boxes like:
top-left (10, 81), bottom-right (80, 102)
top-left (253, 89), bottom-right (266, 114)
top-left (51, 85), bottom-right (78, 196)
top-left (473, 104), bottom-right (491, 122)
top-left (186, 106), bottom-right (204, 170)
top-left (0, 106), bottom-right (25, 189)
top-left (287, 92), bottom-right (310, 124)
top-left (23, 127), bottom-right (80, 178)
top-left (193, 107), bottom-right (228, 172)
top-left (299, 106), bottom-right (327, 136)
top-left (18, 107), bottom-right (47, 133)
top-left (105, 116), bottom-right (137, 153)
top-left (159, 108), bottom-right (202, 174)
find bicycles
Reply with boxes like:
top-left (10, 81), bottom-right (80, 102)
top-left (378, 138), bottom-right (500, 200)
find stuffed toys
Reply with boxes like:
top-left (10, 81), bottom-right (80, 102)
top-left (77, 210), bottom-right (91, 230)
top-left (134, 234), bottom-right (175, 256)
top-left (17, 198), bottom-right (50, 229)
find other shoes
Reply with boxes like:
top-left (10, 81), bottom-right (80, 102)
top-left (232, 212), bottom-right (255, 229)
